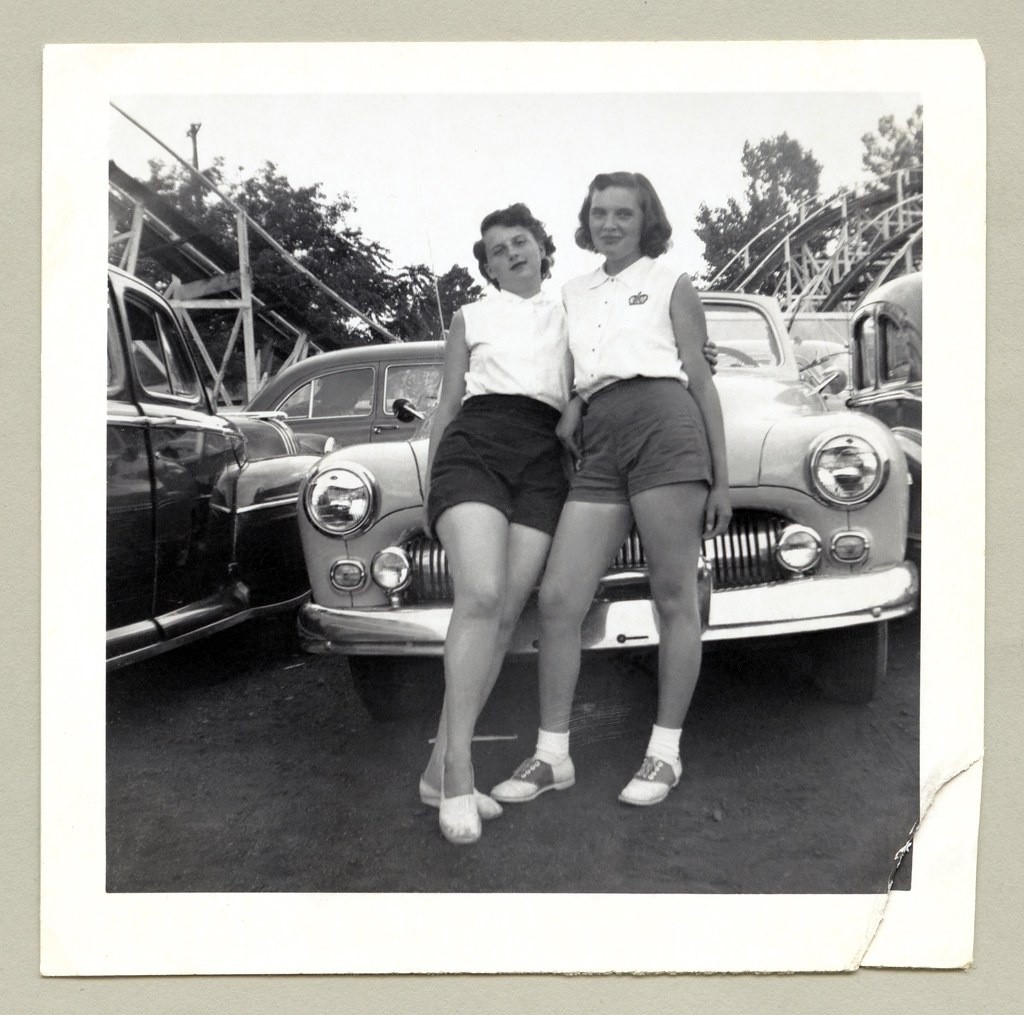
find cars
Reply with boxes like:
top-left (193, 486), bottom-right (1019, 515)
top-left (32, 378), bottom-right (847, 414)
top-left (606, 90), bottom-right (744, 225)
top-left (107, 260), bottom-right (340, 679)
top-left (294, 292), bottom-right (918, 707)
top-left (241, 338), bottom-right (457, 449)
top-left (845, 271), bottom-right (921, 564)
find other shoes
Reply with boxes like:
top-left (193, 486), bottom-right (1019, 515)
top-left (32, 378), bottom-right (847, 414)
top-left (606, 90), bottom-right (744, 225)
top-left (439, 760), bottom-right (483, 845)
top-left (419, 773), bottom-right (504, 821)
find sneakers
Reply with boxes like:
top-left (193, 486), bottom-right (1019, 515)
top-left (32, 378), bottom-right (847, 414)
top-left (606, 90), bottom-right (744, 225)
top-left (617, 748), bottom-right (683, 806)
top-left (489, 753), bottom-right (576, 803)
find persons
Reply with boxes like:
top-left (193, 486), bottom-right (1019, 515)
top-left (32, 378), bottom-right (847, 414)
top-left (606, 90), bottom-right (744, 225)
top-left (491, 171), bottom-right (732, 805)
top-left (420, 203), bottom-right (718, 843)
